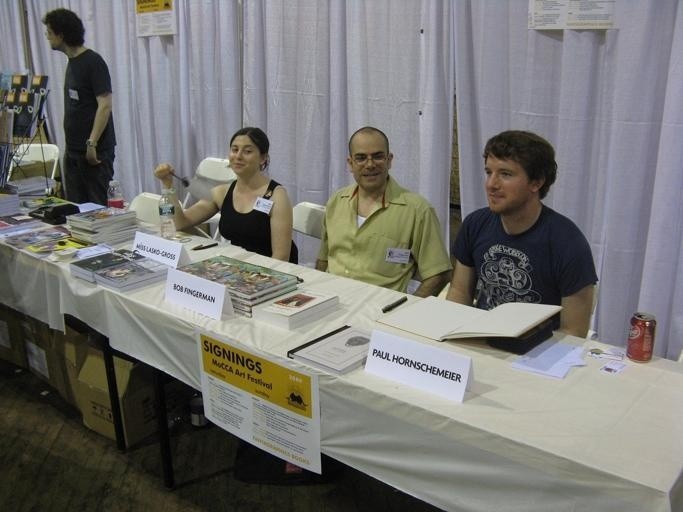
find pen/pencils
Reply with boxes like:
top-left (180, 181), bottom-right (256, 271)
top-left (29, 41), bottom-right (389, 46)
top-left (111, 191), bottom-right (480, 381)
top-left (192, 242), bottom-right (221, 250)
top-left (381, 295), bottom-right (410, 314)
top-left (170, 170), bottom-right (191, 188)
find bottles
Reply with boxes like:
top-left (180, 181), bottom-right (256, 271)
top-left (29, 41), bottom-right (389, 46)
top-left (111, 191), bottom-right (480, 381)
top-left (627, 312), bottom-right (657, 365)
top-left (106, 180), bottom-right (125, 209)
top-left (157, 187), bottom-right (175, 237)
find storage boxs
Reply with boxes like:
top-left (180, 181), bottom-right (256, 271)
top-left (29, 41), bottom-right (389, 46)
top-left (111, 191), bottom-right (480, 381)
top-left (0, 302), bottom-right (21, 374)
top-left (18, 314), bottom-right (91, 405)
top-left (75, 343), bottom-right (158, 450)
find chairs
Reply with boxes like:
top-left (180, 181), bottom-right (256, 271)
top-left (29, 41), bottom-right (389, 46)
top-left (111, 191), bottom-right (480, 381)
top-left (128, 192), bottom-right (184, 232)
top-left (289, 200), bottom-right (328, 240)
top-left (4, 142), bottom-right (59, 196)
top-left (180, 156), bottom-right (238, 240)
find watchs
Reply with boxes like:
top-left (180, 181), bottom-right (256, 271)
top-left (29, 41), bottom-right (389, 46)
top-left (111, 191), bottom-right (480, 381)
top-left (86, 139), bottom-right (97, 146)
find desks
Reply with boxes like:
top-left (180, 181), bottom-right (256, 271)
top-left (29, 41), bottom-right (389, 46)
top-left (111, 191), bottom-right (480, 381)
top-left (0, 197), bottom-right (682, 510)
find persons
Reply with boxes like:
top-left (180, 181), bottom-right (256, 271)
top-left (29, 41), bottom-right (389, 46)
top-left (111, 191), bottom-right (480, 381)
top-left (154, 127), bottom-right (298, 266)
top-left (441, 129), bottom-right (598, 340)
top-left (316, 126), bottom-right (454, 298)
top-left (41, 7), bottom-right (117, 208)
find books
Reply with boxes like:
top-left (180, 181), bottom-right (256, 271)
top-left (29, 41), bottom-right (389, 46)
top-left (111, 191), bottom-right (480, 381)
top-left (248, 304), bottom-right (338, 330)
top-left (376, 295), bottom-right (564, 343)
top-left (233, 306), bottom-right (252, 318)
top-left (5, 176), bottom-right (58, 196)
top-left (68, 249), bottom-right (171, 293)
top-left (10, 74), bottom-right (29, 90)
top-left (176, 255), bottom-right (298, 299)
top-left (31, 75), bottom-right (47, 89)
top-left (288, 326), bottom-right (371, 375)
top-left (0, 218), bottom-right (87, 261)
top-left (224, 284), bottom-right (297, 306)
top-left (252, 289), bottom-right (339, 315)
top-left (1, 89), bottom-right (45, 190)
top-left (0, 196), bottom-right (22, 216)
top-left (66, 207), bottom-right (138, 245)
top-left (231, 300), bottom-right (253, 312)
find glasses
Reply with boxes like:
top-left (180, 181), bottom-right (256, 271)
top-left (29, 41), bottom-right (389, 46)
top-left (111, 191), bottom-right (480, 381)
top-left (351, 154), bottom-right (389, 164)
